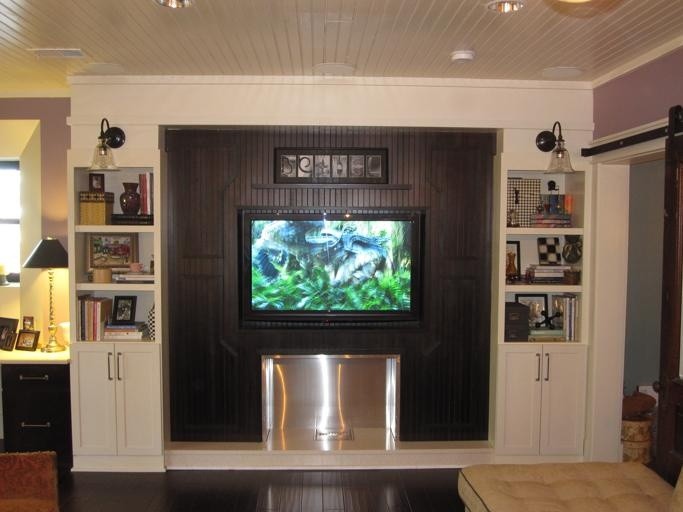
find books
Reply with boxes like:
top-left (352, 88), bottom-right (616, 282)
top-left (525, 214), bottom-right (580, 342)
top-left (73, 170), bottom-right (155, 343)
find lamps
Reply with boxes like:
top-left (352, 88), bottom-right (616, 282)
top-left (22, 236), bottom-right (68, 353)
top-left (85, 118), bottom-right (127, 173)
top-left (535, 121), bottom-right (578, 175)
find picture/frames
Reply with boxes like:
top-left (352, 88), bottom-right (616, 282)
top-left (516, 294), bottom-right (549, 329)
top-left (85, 231), bottom-right (139, 274)
top-left (507, 240), bottom-right (521, 280)
top-left (112, 296), bottom-right (136, 326)
top-left (0, 316), bottom-right (41, 351)
top-left (274, 146), bottom-right (390, 185)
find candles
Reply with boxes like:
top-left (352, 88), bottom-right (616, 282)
top-left (0, 263), bottom-right (7, 275)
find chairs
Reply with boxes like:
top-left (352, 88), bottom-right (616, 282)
top-left (456, 462), bottom-right (683, 512)
top-left (0, 451), bottom-right (61, 512)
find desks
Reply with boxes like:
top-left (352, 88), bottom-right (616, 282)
top-left (0, 347), bottom-right (72, 485)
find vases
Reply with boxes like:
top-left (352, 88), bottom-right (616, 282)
top-left (119, 182), bottom-right (140, 215)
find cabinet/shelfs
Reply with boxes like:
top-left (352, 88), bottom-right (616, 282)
top-left (65, 149), bottom-right (169, 473)
top-left (489, 154), bottom-right (589, 461)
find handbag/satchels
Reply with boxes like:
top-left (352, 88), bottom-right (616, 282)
top-left (621, 391), bottom-right (657, 418)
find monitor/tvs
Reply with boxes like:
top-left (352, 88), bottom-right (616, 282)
top-left (242, 213), bottom-right (420, 322)
